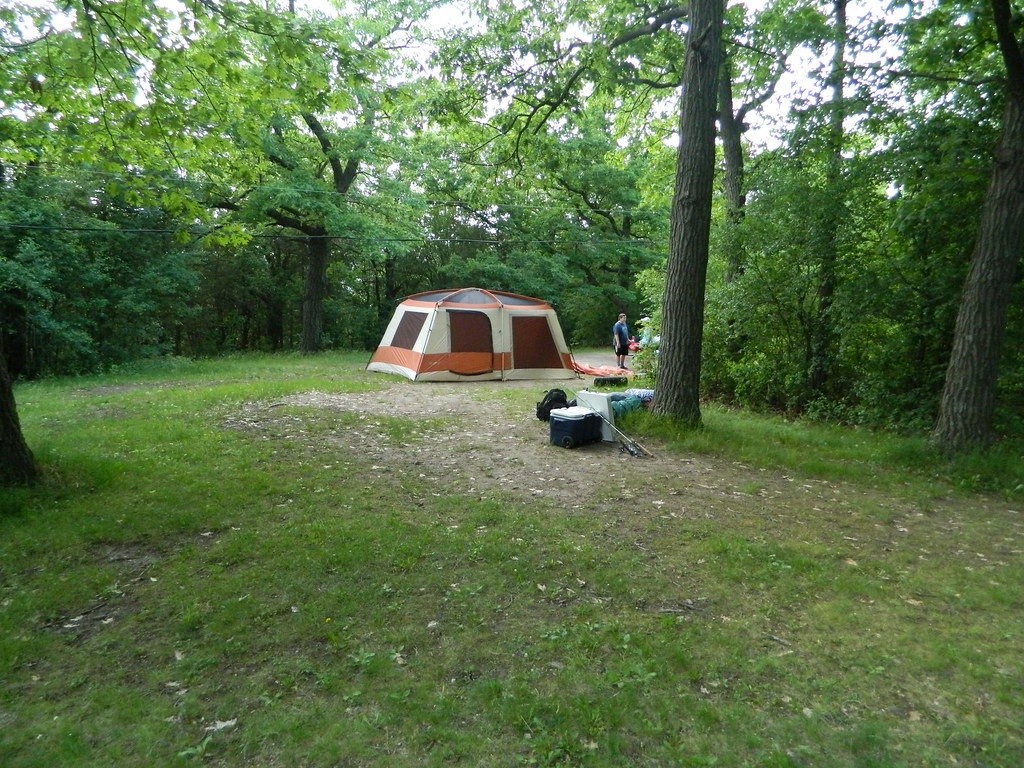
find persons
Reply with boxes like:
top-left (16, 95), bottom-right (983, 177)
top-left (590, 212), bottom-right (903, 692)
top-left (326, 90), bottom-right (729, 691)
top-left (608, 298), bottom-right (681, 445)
top-left (635, 316), bottom-right (656, 349)
top-left (612, 312), bottom-right (635, 370)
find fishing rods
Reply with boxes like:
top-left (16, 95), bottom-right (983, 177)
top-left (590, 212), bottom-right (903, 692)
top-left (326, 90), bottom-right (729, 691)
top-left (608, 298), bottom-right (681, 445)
top-left (566, 386), bottom-right (654, 459)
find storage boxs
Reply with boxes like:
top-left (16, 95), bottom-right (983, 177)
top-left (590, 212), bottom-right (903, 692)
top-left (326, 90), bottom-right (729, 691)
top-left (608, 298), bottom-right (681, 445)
top-left (550, 406), bottom-right (602, 450)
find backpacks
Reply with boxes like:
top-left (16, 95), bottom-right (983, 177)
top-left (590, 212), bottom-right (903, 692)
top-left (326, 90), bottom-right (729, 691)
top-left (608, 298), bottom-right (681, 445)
top-left (536, 388), bottom-right (567, 422)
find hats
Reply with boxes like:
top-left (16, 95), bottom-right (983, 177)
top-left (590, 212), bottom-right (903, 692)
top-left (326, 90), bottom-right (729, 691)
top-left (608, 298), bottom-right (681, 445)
top-left (641, 316), bottom-right (651, 322)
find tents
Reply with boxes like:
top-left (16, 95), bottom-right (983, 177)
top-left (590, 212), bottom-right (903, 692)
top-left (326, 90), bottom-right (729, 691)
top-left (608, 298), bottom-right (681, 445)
top-left (364, 287), bottom-right (581, 382)
